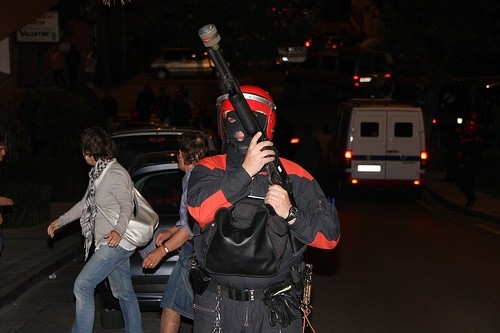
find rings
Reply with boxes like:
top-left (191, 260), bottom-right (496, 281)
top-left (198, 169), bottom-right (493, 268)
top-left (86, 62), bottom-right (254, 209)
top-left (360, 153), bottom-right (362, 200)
top-left (150, 263), bottom-right (153, 266)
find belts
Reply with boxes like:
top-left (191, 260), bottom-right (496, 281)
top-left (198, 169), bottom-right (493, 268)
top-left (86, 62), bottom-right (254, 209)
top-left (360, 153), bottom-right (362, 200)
top-left (208, 282), bottom-right (265, 302)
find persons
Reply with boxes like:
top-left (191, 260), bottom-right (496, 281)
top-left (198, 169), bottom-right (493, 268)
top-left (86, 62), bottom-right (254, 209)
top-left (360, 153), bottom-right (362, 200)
top-left (439, 121), bottom-right (480, 206)
top-left (101, 88), bottom-right (118, 118)
top-left (46, 126), bottom-right (147, 333)
top-left (137, 84), bottom-right (192, 128)
top-left (142, 132), bottom-right (216, 332)
top-left (0, 126), bottom-right (14, 265)
top-left (187, 73), bottom-right (342, 333)
top-left (271, 120), bottom-right (337, 272)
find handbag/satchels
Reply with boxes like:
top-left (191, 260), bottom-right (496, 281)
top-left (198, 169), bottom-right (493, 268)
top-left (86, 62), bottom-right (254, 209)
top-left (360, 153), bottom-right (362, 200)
top-left (98, 188), bottom-right (159, 248)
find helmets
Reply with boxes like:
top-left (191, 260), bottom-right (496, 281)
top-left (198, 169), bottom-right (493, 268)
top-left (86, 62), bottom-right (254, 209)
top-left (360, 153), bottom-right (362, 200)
top-left (216, 85), bottom-right (276, 141)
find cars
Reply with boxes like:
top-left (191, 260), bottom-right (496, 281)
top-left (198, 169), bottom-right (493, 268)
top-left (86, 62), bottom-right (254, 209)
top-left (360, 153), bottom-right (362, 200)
top-left (110, 129), bottom-right (220, 176)
top-left (103, 162), bottom-right (191, 309)
top-left (149, 48), bottom-right (222, 81)
top-left (256, 37), bottom-right (308, 66)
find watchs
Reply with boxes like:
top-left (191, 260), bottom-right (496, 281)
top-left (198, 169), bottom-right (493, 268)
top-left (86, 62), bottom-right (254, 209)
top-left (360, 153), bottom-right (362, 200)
top-left (284, 205), bottom-right (298, 222)
top-left (161, 243), bottom-right (170, 255)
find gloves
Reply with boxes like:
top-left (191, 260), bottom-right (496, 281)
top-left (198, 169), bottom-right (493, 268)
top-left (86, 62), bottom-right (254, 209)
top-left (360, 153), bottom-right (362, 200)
top-left (263, 281), bottom-right (300, 327)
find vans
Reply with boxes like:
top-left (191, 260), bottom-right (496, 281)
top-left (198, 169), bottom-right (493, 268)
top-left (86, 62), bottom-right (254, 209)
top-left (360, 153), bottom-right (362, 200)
top-left (337, 98), bottom-right (427, 200)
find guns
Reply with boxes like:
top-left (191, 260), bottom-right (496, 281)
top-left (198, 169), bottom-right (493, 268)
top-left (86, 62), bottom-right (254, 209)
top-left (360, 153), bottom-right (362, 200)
top-left (199, 24), bottom-right (308, 258)
